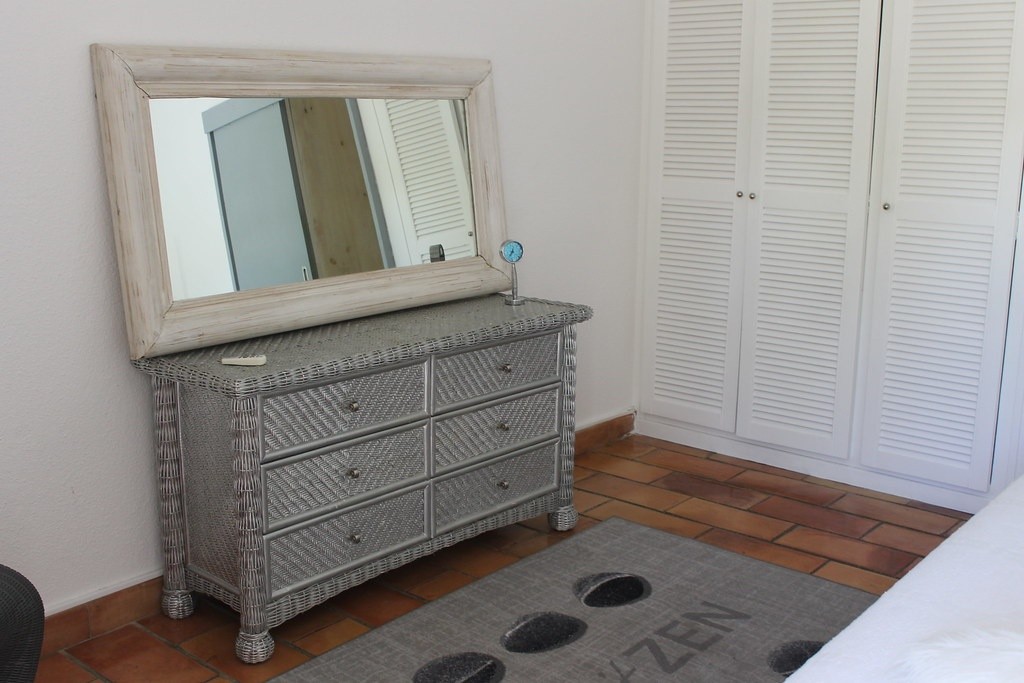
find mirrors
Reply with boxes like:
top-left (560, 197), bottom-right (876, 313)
top-left (88, 42), bottom-right (514, 356)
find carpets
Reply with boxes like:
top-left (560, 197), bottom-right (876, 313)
top-left (266, 516), bottom-right (879, 683)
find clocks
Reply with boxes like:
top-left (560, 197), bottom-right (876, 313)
top-left (501, 241), bottom-right (525, 305)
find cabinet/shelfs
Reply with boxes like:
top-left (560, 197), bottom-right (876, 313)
top-left (633, 0), bottom-right (1024, 516)
top-left (356, 99), bottom-right (479, 271)
top-left (127, 294), bottom-right (592, 663)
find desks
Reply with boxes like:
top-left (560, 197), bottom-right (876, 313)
top-left (775, 471), bottom-right (1024, 683)
